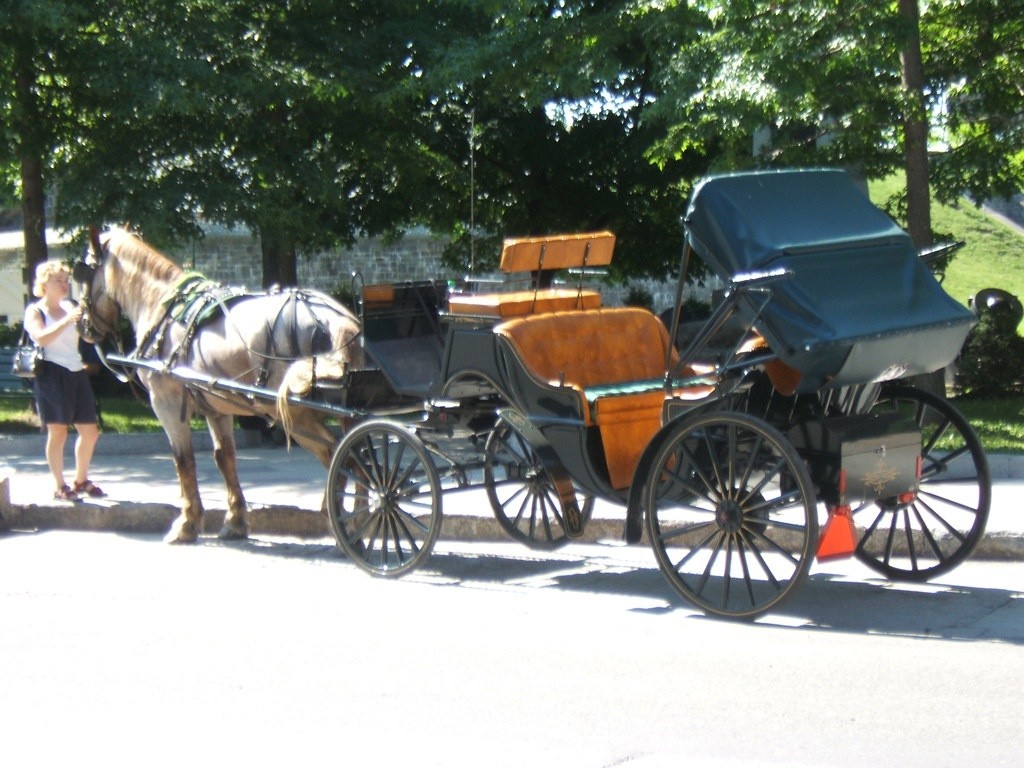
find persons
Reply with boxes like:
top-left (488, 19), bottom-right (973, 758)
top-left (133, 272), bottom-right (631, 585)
top-left (22, 259), bottom-right (108, 502)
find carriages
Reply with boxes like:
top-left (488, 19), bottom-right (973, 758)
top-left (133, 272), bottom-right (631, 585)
top-left (77, 164), bottom-right (994, 623)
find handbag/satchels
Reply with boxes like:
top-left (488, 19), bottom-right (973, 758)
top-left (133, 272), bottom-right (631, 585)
top-left (9, 306), bottom-right (47, 377)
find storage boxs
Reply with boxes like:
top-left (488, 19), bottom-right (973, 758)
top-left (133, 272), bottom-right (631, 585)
top-left (780, 412), bottom-right (922, 506)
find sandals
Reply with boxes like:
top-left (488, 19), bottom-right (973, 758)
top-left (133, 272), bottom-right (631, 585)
top-left (73, 479), bottom-right (107, 498)
top-left (53, 484), bottom-right (82, 503)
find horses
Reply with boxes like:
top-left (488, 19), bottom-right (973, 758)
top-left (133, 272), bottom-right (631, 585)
top-left (75, 217), bottom-right (374, 560)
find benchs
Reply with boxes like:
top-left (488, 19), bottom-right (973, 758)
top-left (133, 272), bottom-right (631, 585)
top-left (492, 306), bottom-right (715, 490)
top-left (660, 335), bottom-right (883, 444)
top-left (438, 231), bottom-right (616, 332)
top-left (0, 347), bottom-right (103, 428)
